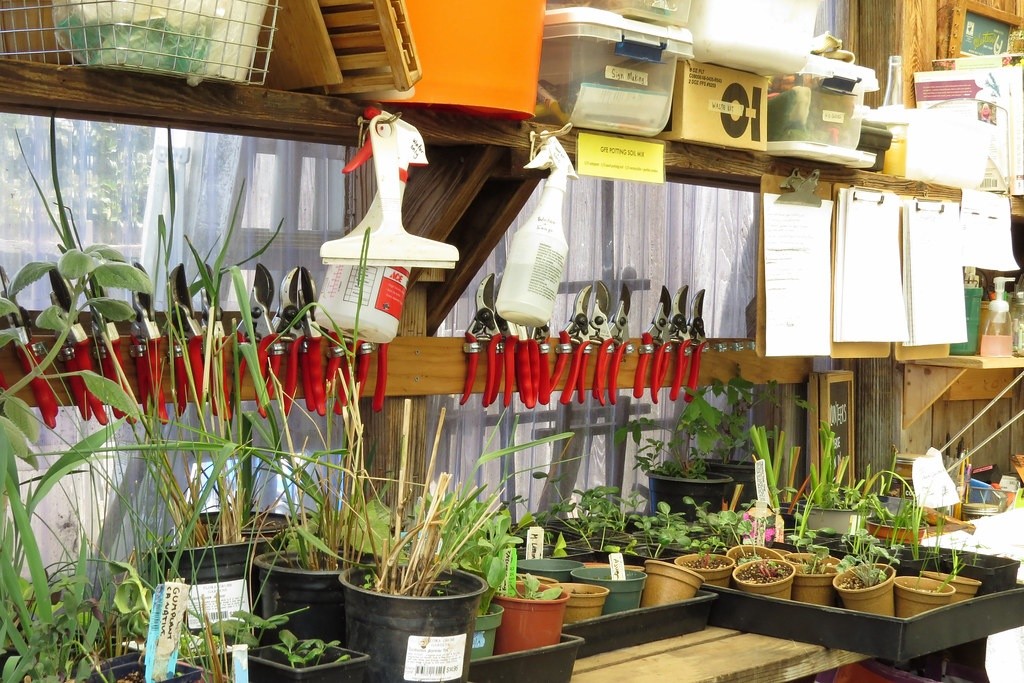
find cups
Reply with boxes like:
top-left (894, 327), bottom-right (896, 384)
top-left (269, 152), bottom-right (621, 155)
top-left (949, 288), bottom-right (983, 355)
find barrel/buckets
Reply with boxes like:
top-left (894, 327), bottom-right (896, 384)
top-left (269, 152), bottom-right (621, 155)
top-left (961, 502), bottom-right (1000, 520)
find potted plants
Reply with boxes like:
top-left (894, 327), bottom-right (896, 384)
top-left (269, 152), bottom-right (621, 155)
top-left (0, 111), bottom-right (1020, 683)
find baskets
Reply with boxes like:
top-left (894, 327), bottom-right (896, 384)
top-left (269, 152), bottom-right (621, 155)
top-left (0, 0), bottom-right (284, 85)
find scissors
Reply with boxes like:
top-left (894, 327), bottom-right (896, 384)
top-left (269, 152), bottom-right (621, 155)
top-left (0, 261), bottom-right (388, 429)
top-left (459, 271), bottom-right (706, 410)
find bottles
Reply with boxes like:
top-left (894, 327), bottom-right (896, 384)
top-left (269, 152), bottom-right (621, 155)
top-left (882, 56), bottom-right (907, 176)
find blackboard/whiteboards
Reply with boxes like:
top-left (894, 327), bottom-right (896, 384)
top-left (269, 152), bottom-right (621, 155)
top-left (806, 370), bottom-right (857, 496)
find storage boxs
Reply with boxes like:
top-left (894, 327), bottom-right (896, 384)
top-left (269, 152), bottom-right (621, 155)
top-left (542, 9), bottom-right (880, 154)
